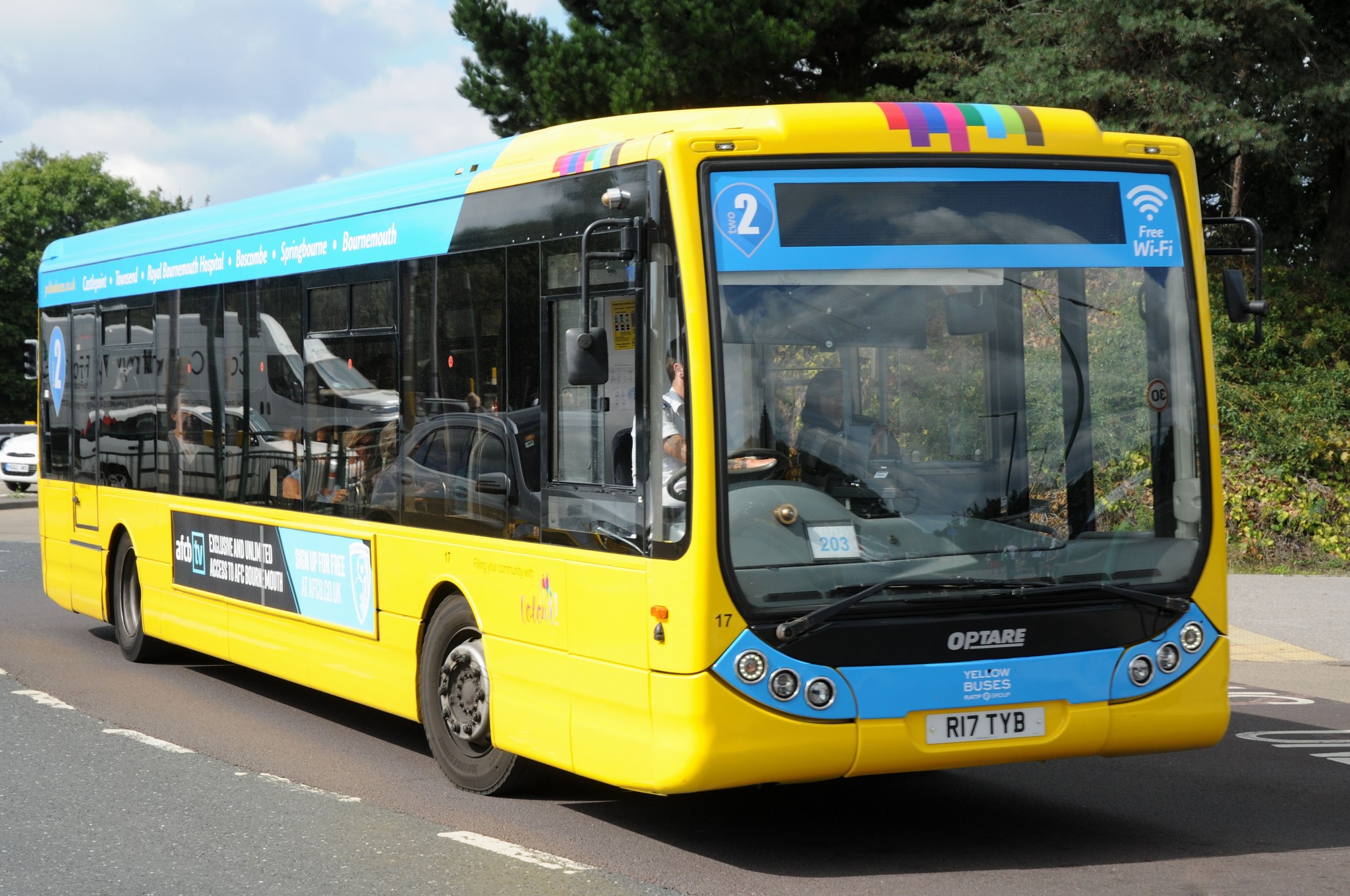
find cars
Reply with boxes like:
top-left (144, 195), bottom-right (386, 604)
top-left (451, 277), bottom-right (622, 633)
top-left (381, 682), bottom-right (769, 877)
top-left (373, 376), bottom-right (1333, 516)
top-left (0, 434), bottom-right (41, 493)
top-left (79, 398), bottom-right (365, 503)
top-left (375, 405), bottom-right (564, 522)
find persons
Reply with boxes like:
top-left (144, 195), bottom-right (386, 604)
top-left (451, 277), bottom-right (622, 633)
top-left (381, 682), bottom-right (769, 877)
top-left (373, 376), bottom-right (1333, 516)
top-left (797, 366), bottom-right (900, 520)
top-left (628, 332), bottom-right (776, 534)
top-left (160, 384), bottom-right (482, 515)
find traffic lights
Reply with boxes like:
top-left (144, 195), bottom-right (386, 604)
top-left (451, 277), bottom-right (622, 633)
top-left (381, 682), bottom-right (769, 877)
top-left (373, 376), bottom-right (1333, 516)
top-left (22, 338), bottom-right (40, 381)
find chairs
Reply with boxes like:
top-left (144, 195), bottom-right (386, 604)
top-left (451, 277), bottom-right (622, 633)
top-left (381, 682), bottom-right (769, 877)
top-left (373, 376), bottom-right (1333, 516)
top-left (612, 428), bottom-right (634, 488)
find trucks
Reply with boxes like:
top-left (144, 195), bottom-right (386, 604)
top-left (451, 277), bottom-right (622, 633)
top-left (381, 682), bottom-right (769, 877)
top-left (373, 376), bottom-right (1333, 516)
top-left (39, 309), bottom-right (400, 476)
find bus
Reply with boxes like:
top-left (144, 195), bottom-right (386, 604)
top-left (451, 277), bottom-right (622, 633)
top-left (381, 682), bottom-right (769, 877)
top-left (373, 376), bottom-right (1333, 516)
top-left (38, 97), bottom-right (1236, 809)
